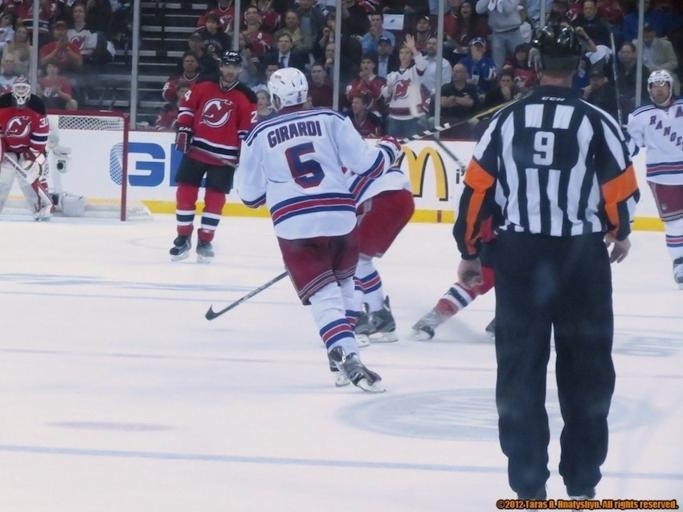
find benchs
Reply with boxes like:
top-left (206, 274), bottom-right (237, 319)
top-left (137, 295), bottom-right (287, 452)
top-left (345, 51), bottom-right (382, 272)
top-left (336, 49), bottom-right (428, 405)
top-left (0, 0), bottom-right (683, 146)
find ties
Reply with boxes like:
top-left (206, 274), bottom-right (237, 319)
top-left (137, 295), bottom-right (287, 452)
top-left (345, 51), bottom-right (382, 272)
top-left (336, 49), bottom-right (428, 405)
top-left (279, 56), bottom-right (285, 67)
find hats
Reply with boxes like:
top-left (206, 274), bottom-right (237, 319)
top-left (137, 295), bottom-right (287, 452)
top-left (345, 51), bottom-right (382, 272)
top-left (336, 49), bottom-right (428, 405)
top-left (377, 15), bottom-right (487, 47)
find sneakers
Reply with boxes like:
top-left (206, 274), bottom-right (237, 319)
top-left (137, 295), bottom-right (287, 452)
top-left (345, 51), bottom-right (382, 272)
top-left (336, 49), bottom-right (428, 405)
top-left (327, 345), bottom-right (343, 371)
top-left (170, 234), bottom-right (191, 255)
top-left (485, 317), bottom-right (496, 333)
top-left (411, 319), bottom-right (434, 339)
top-left (196, 239), bottom-right (214, 257)
top-left (368, 296), bottom-right (395, 335)
top-left (673, 257), bottom-right (683, 283)
top-left (343, 352), bottom-right (382, 391)
top-left (353, 295), bottom-right (370, 335)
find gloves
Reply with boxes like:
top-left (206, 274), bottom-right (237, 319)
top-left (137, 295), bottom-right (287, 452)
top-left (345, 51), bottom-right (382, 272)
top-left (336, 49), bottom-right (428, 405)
top-left (376, 135), bottom-right (404, 167)
top-left (24, 160), bottom-right (42, 186)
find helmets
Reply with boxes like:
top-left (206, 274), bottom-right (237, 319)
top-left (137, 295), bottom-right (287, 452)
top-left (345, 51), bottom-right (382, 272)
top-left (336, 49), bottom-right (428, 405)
top-left (219, 50), bottom-right (244, 73)
top-left (267, 67), bottom-right (309, 112)
top-left (12, 76), bottom-right (31, 86)
top-left (647, 69), bottom-right (674, 82)
top-left (526, 24), bottom-right (582, 70)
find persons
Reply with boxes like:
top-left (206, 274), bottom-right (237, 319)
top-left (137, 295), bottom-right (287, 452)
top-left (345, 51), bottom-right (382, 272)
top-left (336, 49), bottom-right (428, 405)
top-left (453, 24), bottom-right (640, 510)
top-left (624, 69), bottom-right (682, 283)
top-left (409, 214), bottom-right (496, 341)
top-left (0, 1), bottom-right (134, 112)
top-left (340, 135), bottom-right (414, 334)
top-left (167, 50), bottom-right (251, 257)
top-left (153, 1), bottom-right (681, 141)
top-left (234, 68), bottom-right (386, 387)
top-left (0, 77), bottom-right (55, 222)
